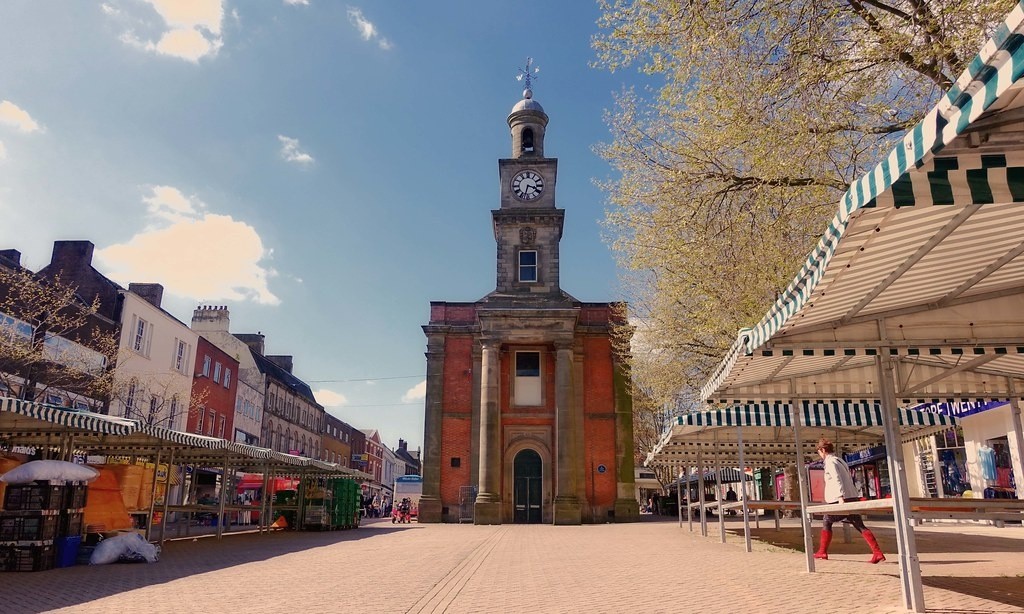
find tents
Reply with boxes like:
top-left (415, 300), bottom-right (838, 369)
top-left (641, 0), bottom-right (1024, 613)
top-left (0, 395), bottom-right (374, 549)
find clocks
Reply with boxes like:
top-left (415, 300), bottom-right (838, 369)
top-left (509, 168), bottom-right (546, 203)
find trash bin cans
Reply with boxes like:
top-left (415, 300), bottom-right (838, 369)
top-left (53, 535), bottom-right (82, 568)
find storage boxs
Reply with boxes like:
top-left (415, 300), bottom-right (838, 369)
top-left (0, 479), bottom-right (90, 571)
top-left (275, 477), bottom-right (362, 531)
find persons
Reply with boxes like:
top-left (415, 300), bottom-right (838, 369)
top-left (642, 492), bottom-right (661, 515)
top-left (812, 438), bottom-right (886, 564)
top-left (977, 444), bottom-right (998, 480)
top-left (360, 491), bottom-right (412, 523)
top-left (726, 486), bottom-right (737, 515)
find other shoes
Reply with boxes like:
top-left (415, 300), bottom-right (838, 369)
top-left (726, 514), bottom-right (729, 515)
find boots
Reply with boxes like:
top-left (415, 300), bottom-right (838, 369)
top-left (861, 529), bottom-right (886, 564)
top-left (813, 530), bottom-right (833, 560)
